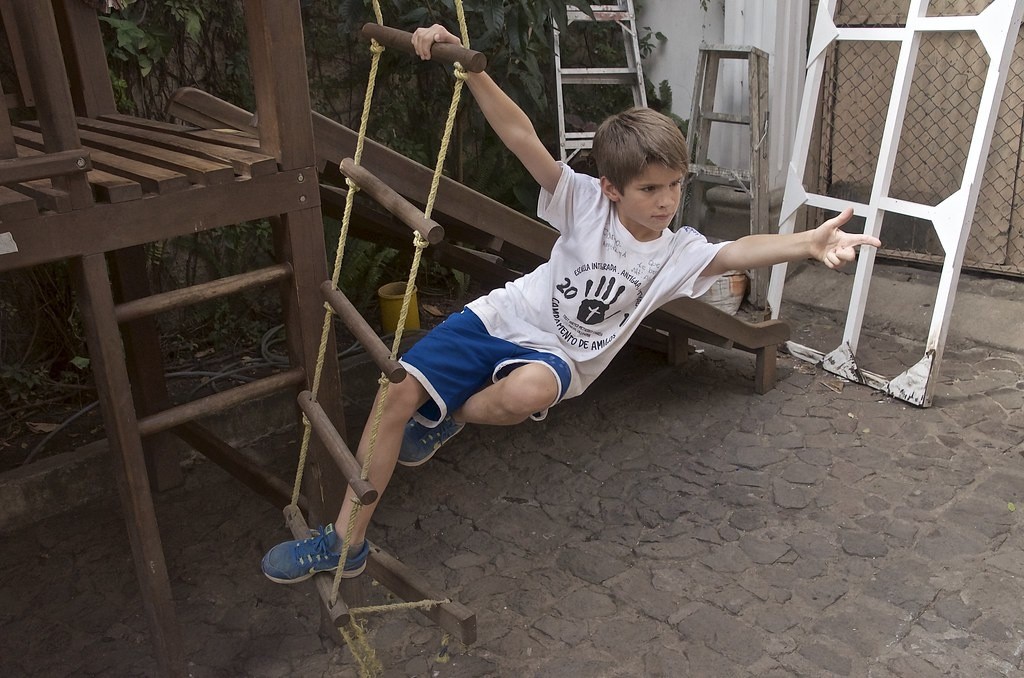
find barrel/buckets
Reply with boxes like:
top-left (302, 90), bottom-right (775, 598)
top-left (377, 282), bottom-right (420, 333)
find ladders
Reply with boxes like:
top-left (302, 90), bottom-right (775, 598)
top-left (550, 0), bottom-right (648, 166)
top-left (670, 41), bottom-right (770, 310)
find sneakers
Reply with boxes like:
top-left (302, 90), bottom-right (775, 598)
top-left (261, 522), bottom-right (370, 584)
top-left (397, 412), bottom-right (466, 466)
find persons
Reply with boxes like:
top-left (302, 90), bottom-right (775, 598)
top-left (258, 22), bottom-right (883, 584)
